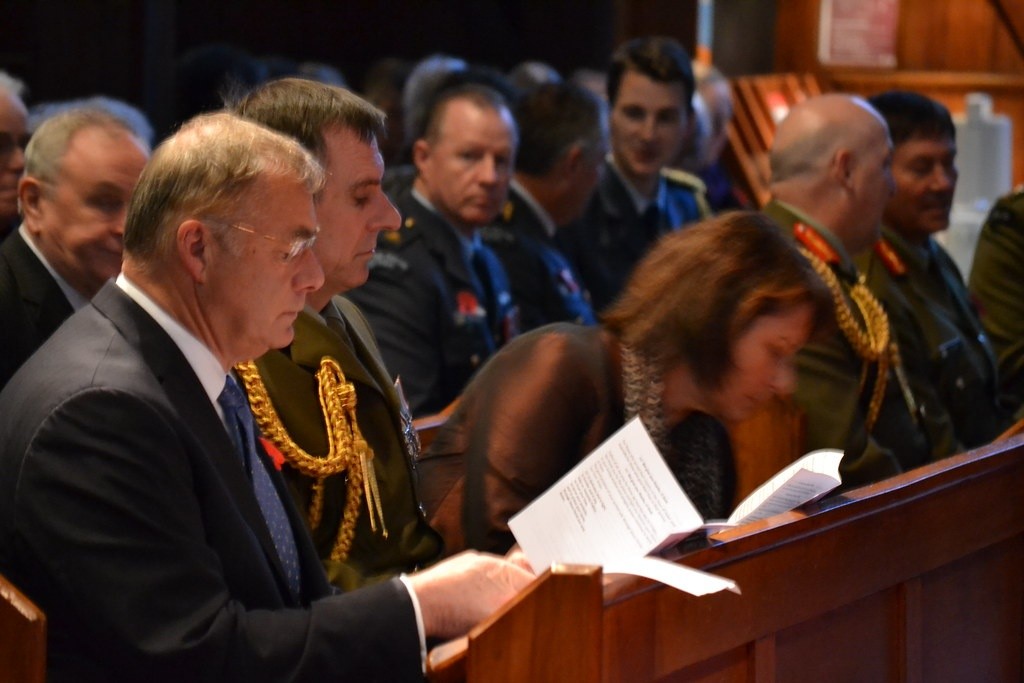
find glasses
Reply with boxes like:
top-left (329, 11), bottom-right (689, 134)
top-left (197, 216), bottom-right (319, 261)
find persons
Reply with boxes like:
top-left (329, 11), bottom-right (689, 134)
top-left (1, 107), bottom-right (533, 683)
top-left (0, 26), bottom-right (752, 419)
top-left (970, 186), bottom-right (1024, 427)
top-left (420, 209), bottom-right (822, 564)
top-left (227, 78), bottom-right (451, 596)
top-left (757, 89), bottom-right (966, 491)
top-left (854, 93), bottom-right (1014, 451)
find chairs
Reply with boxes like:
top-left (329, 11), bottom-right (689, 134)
top-left (724, 69), bottom-right (822, 209)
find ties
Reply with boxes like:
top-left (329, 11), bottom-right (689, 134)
top-left (217, 374), bottom-right (300, 596)
top-left (469, 250), bottom-right (501, 346)
top-left (637, 202), bottom-right (659, 250)
top-left (326, 316), bottom-right (356, 357)
